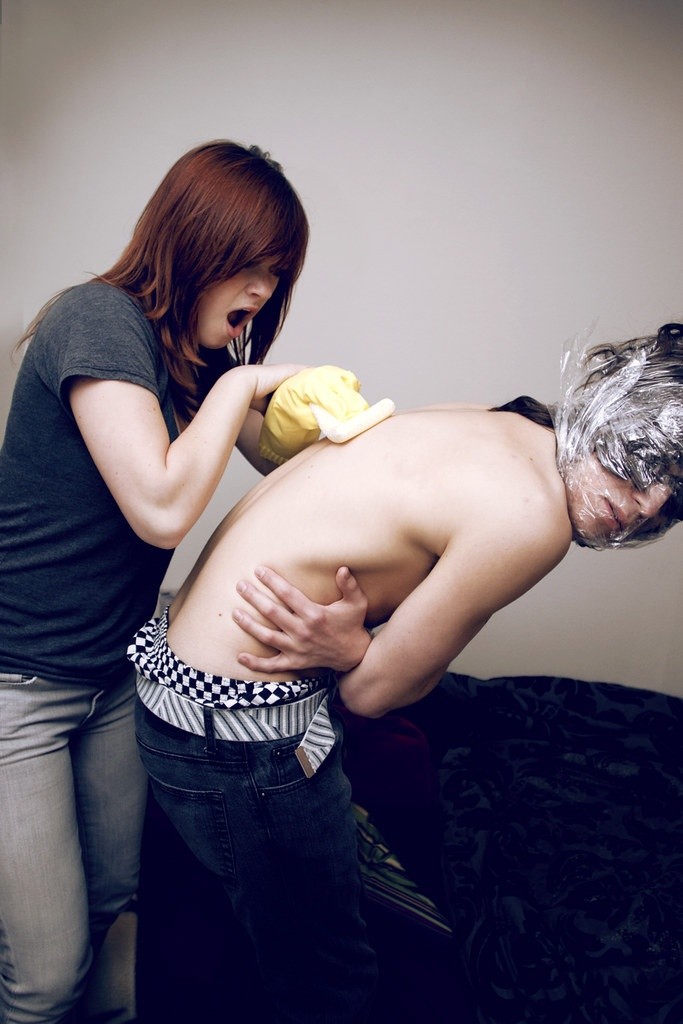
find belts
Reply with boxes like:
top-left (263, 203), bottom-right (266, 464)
top-left (135, 672), bottom-right (337, 778)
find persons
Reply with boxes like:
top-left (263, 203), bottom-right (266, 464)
top-left (125, 321), bottom-right (683, 1024)
top-left (0, 141), bottom-right (369, 1024)
